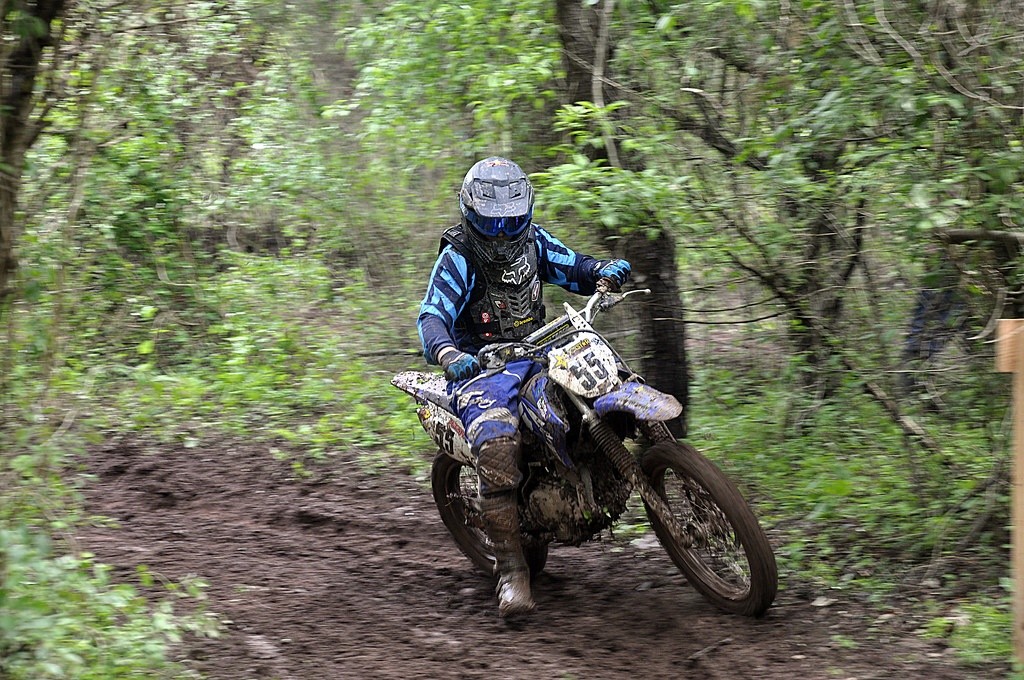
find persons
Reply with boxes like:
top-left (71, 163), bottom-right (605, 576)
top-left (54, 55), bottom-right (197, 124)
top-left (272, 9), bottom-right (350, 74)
top-left (417, 155), bottom-right (634, 619)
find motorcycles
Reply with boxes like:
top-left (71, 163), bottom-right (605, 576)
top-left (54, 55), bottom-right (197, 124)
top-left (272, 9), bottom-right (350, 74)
top-left (390, 279), bottom-right (778, 620)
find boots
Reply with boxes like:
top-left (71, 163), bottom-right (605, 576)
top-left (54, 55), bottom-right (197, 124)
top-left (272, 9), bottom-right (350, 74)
top-left (476, 494), bottom-right (538, 622)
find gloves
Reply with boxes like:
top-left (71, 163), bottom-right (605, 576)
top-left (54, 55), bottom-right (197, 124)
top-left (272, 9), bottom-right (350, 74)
top-left (579, 258), bottom-right (632, 293)
top-left (439, 350), bottom-right (482, 383)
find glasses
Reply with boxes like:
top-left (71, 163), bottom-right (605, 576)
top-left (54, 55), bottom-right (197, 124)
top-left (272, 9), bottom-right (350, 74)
top-left (472, 214), bottom-right (530, 237)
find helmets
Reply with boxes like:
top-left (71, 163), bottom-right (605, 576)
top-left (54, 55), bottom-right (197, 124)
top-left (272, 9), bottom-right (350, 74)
top-left (457, 156), bottom-right (535, 270)
